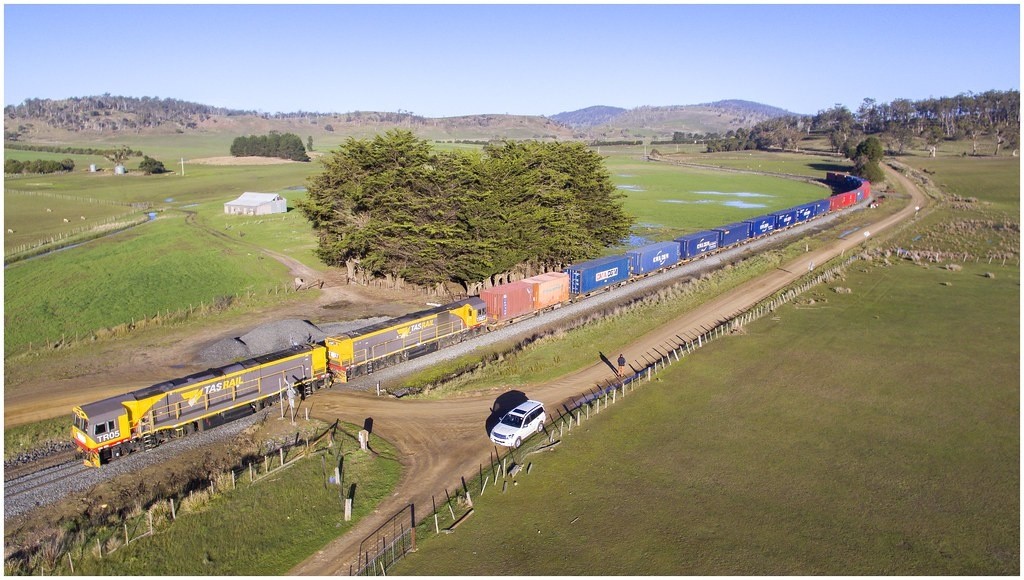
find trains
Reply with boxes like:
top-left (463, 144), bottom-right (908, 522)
top-left (70, 171), bottom-right (871, 467)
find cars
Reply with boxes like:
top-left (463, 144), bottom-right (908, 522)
top-left (489, 400), bottom-right (547, 449)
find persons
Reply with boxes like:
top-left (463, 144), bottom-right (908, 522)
top-left (617, 353), bottom-right (626, 378)
